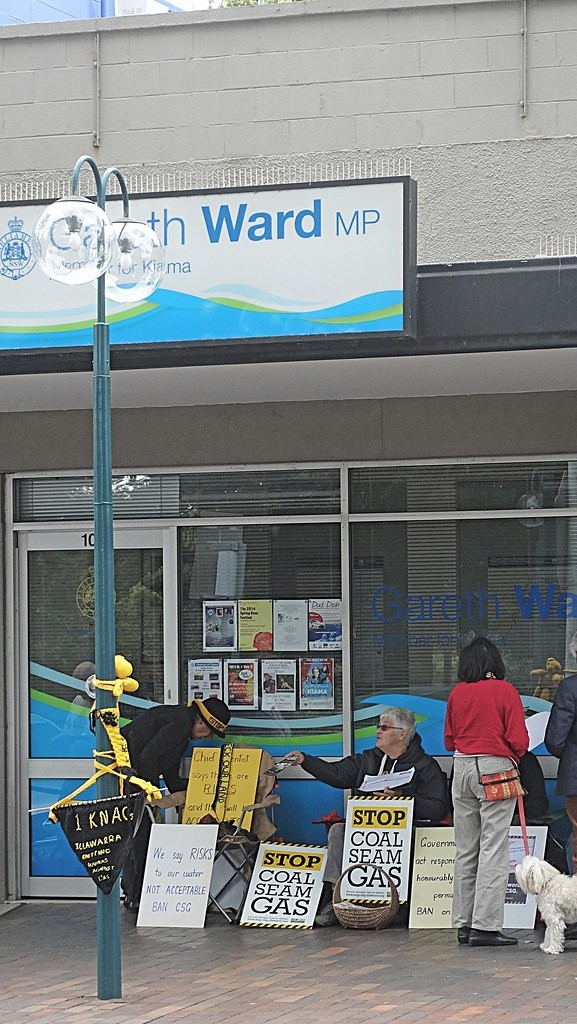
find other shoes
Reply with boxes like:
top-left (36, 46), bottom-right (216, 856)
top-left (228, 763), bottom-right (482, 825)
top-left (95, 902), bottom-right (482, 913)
top-left (314, 901), bottom-right (338, 926)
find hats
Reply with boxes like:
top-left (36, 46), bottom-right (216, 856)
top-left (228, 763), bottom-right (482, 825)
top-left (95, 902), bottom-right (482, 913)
top-left (190, 698), bottom-right (231, 739)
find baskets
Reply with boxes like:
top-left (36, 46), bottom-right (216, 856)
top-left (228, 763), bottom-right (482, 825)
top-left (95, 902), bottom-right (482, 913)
top-left (332, 864), bottom-right (400, 929)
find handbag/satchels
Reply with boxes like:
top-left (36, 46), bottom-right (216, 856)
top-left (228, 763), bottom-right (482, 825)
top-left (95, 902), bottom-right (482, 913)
top-left (480, 769), bottom-right (524, 801)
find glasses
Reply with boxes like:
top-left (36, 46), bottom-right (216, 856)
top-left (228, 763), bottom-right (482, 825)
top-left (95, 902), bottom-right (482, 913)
top-left (376, 724), bottom-right (405, 732)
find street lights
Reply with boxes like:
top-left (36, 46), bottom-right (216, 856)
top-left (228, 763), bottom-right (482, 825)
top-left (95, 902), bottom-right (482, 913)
top-left (34, 154), bottom-right (166, 1000)
top-left (518, 465), bottom-right (555, 675)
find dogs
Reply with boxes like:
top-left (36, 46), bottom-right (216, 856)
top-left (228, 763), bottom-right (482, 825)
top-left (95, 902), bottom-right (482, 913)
top-left (515, 855), bottom-right (577, 955)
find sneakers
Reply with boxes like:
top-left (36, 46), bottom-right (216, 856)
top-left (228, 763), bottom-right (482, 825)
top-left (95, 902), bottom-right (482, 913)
top-left (458, 928), bottom-right (471, 943)
top-left (468, 927), bottom-right (518, 945)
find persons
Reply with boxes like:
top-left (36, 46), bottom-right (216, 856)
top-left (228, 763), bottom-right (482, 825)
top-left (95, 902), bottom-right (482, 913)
top-left (263, 673), bottom-right (274, 693)
top-left (449, 752), bottom-right (549, 826)
top-left (118, 698), bottom-right (230, 914)
top-left (282, 707), bottom-right (447, 927)
top-left (62, 662), bottom-right (95, 740)
top-left (545, 630), bottom-right (577, 941)
top-left (444, 637), bottom-right (529, 945)
top-left (311, 667), bottom-right (326, 684)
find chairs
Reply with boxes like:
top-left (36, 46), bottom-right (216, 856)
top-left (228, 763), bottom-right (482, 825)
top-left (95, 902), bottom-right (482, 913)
top-left (151, 745), bottom-right (280, 925)
top-left (312, 772), bottom-right (453, 914)
top-left (528, 808), bottom-right (567, 870)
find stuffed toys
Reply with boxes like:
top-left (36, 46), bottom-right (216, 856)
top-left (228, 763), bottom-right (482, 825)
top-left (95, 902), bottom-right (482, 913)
top-left (531, 656), bottom-right (565, 701)
